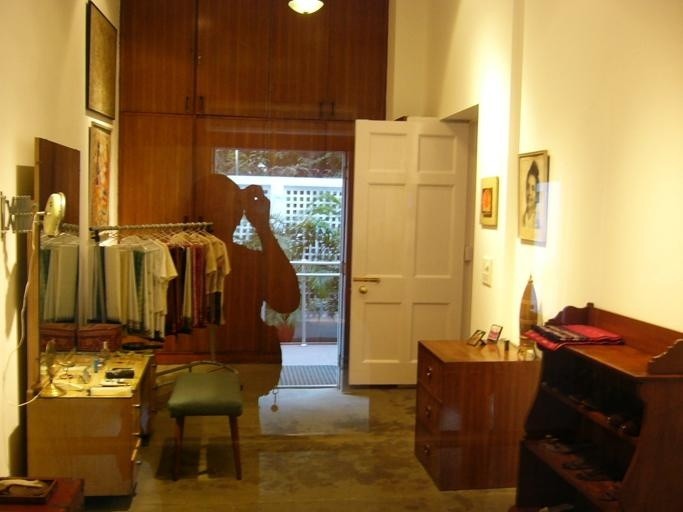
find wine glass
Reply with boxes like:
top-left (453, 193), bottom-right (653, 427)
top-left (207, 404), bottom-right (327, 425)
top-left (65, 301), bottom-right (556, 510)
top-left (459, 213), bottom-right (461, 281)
top-left (57, 346), bottom-right (78, 379)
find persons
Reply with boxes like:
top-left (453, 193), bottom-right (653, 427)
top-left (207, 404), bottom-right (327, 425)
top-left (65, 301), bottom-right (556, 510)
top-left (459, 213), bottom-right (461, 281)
top-left (520, 160), bottom-right (542, 236)
top-left (179, 173), bottom-right (301, 512)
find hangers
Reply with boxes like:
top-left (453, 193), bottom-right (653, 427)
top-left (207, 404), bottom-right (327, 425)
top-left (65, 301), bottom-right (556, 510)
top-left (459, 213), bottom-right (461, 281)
top-left (108, 221), bottom-right (213, 245)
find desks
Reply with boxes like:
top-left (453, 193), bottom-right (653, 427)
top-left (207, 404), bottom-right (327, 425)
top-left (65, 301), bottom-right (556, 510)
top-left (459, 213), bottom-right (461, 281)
top-left (1, 473), bottom-right (89, 512)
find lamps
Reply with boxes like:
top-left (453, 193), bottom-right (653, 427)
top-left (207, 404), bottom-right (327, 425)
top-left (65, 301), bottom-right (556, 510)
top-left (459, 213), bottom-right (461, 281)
top-left (288, 0), bottom-right (323, 15)
top-left (0, 189), bottom-right (66, 243)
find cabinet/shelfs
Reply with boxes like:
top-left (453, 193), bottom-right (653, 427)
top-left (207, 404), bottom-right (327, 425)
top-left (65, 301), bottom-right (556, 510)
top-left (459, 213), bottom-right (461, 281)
top-left (119, 0), bottom-right (270, 120)
top-left (272, 0), bottom-right (390, 124)
top-left (512, 300), bottom-right (680, 510)
top-left (26, 348), bottom-right (164, 500)
top-left (412, 336), bottom-right (543, 492)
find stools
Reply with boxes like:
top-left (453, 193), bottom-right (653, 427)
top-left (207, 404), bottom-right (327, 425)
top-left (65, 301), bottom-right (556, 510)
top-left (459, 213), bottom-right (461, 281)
top-left (166, 368), bottom-right (246, 484)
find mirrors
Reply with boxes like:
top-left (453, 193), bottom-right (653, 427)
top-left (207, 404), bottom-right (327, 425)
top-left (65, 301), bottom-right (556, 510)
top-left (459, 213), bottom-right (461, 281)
top-left (27, 136), bottom-right (82, 386)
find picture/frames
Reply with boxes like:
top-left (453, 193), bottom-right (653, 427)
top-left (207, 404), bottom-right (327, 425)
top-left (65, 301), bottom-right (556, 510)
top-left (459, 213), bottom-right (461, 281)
top-left (86, 0), bottom-right (118, 119)
top-left (517, 149), bottom-right (549, 246)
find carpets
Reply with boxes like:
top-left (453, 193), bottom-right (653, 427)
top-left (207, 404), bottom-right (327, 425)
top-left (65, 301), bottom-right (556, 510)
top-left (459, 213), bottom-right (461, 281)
top-left (276, 363), bottom-right (336, 386)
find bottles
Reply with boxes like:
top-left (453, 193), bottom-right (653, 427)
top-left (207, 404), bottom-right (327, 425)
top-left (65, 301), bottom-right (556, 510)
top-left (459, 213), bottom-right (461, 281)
top-left (93, 340), bottom-right (111, 373)
